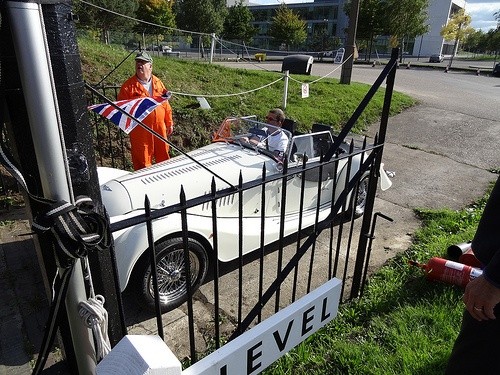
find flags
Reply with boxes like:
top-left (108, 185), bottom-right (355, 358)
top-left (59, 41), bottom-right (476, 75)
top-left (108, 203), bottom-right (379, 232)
top-left (87, 92), bottom-right (170, 135)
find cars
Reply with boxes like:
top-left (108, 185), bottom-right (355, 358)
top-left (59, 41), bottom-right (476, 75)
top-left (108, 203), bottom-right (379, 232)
top-left (430, 54), bottom-right (445, 63)
top-left (95, 112), bottom-right (382, 313)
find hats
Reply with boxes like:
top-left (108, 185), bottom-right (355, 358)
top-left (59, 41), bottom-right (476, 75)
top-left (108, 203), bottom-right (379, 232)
top-left (134, 51), bottom-right (153, 63)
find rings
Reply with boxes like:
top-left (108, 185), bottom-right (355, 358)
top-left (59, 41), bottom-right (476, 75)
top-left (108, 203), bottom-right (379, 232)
top-left (473, 306), bottom-right (483, 311)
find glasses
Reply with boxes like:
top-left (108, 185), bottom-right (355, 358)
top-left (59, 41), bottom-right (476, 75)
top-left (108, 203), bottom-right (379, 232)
top-left (266, 116), bottom-right (278, 122)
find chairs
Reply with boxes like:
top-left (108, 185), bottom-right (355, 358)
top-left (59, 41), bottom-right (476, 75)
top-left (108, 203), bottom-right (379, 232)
top-left (312, 123), bottom-right (330, 133)
top-left (281, 118), bottom-right (294, 137)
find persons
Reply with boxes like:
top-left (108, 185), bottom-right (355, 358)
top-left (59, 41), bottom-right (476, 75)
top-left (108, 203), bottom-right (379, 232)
top-left (446, 175), bottom-right (500, 375)
top-left (240, 108), bottom-right (288, 159)
top-left (117, 52), bottom-right (173, 171)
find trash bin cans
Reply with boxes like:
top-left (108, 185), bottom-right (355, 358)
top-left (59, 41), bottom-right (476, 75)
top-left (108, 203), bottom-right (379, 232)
top-left (282, 55), bottom-right (313, 74)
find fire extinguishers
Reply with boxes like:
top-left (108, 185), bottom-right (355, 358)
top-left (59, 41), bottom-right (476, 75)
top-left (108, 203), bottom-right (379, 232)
top-left (408, 258), bottom-right (483, 288)
